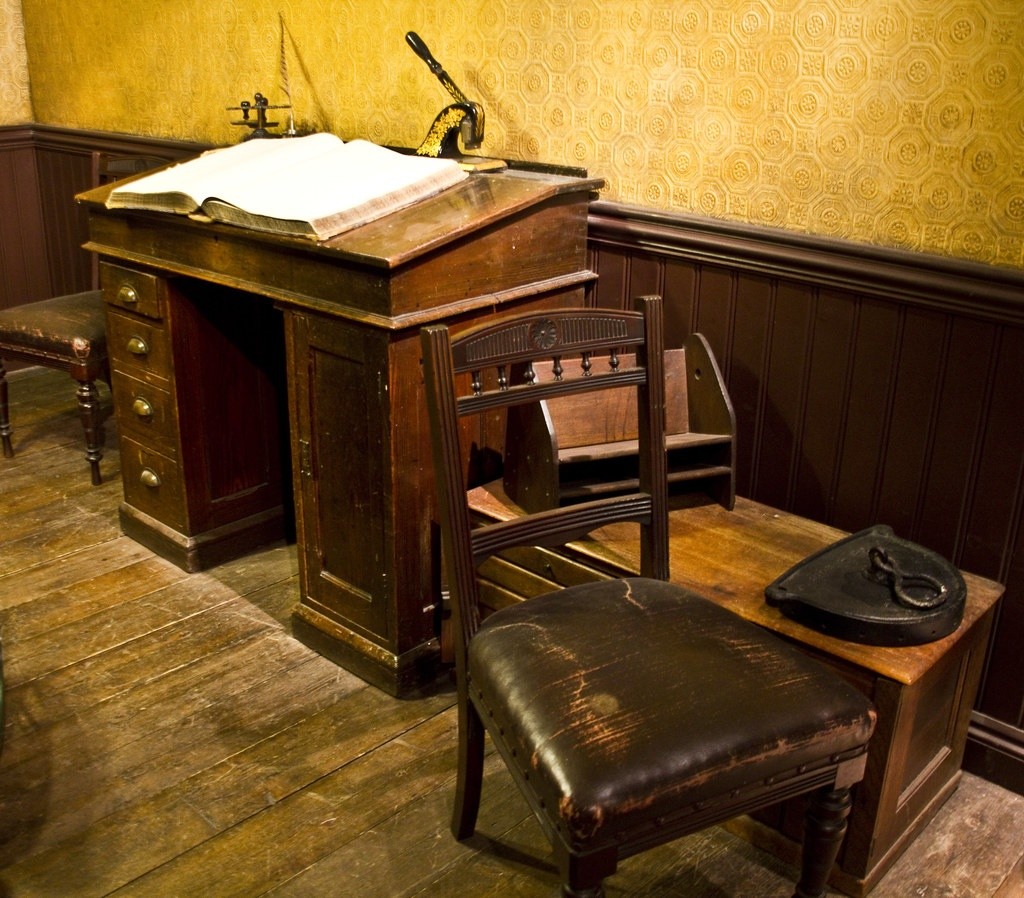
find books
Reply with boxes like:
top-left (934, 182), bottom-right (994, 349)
top-left (105, 131), bottom-right (469, 243)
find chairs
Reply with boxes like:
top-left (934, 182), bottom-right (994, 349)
top-left (0, 151), bottom-right (178, 486)
top-left (420, 295), bottom-right (878, 898)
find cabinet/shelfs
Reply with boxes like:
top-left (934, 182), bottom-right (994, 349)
top-left (437, 474), bottom-right (1006, 898)
top-left (72, 155), bottom-right (606, 702)
